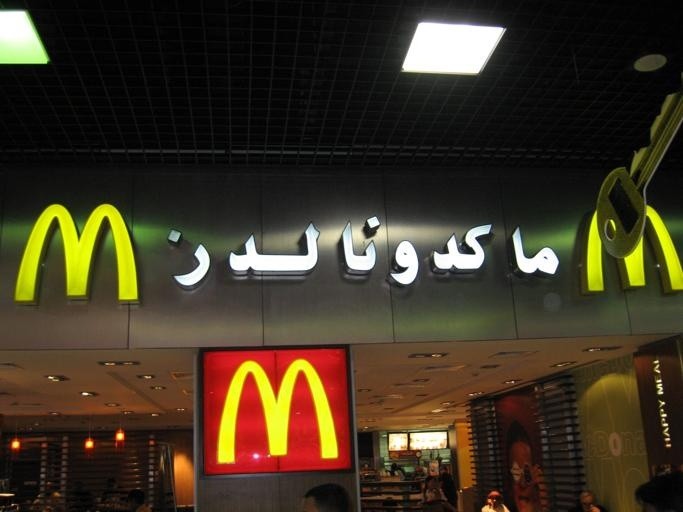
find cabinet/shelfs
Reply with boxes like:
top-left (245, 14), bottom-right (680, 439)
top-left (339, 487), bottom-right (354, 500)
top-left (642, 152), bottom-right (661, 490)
top-left (360, 479), bottom-right (425, 511)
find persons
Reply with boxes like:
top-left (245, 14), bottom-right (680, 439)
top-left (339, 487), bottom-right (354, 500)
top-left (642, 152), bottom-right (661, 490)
top-left (480, 491), bottom-right (510, 512)
top-left (636, 471), bottom-right (683, 511)
top-left (420, 474), bottom-right (448, 502)
top-left (424, 502), bottom-right (455, 512)
top-left (440, 471), bottom-right (457, 507)
top-left (504, 419), bottom-right (538, 511)
top-left (128, 489), bottom-right (152, 512)
top-left (303, 483), bottom-right (349, 512)
top-left (32, 476), bottom-right (128, 512)
top-left (569, 490), bottom-right (605, 512)
